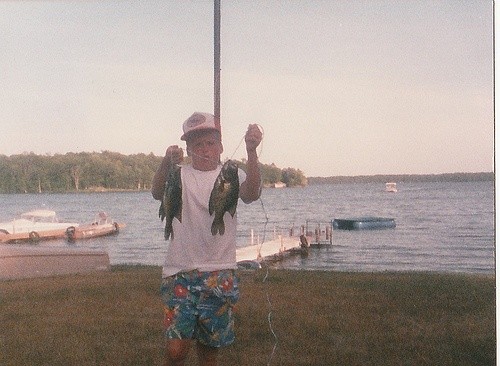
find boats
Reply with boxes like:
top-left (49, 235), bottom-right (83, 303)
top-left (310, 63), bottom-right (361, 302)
top-left (65, 220), bottom-right (119, 240)
top-left (0, 210), bottom-right (79, 241)
top-left (385, 183), bottom-right (397, 192)
top-left (332, 216), bottom-right (396, 231)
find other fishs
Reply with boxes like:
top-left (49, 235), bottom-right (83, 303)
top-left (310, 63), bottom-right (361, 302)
top-left (159, 165), bottom-right (183, 240)
top-left (209, 160), bottom-right (239, 237)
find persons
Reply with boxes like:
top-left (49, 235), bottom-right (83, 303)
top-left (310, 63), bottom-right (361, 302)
top-left (151, 112), bottom-right (263, 366)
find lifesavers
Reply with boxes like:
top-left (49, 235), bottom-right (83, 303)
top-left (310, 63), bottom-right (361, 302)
top-left (67, 226), bottom-right (76, 235)
top-left (29, 231), bottom-right (40, 240)
top-left (113, 222), bottom-right (119, 231)
top-left (300, 235), bottom-right (308, 248)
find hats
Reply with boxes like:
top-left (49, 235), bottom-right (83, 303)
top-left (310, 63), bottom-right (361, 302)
top-left (180, 112), bottom-right (221, 141)
